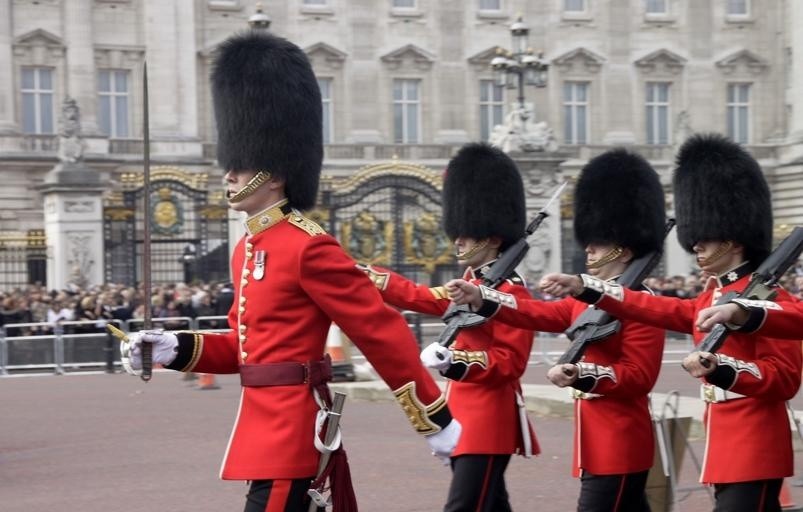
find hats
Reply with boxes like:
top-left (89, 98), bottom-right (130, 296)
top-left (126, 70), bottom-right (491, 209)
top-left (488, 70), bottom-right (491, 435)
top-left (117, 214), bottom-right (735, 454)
top-left (573, 148), bottom-right (665, 270)
top-left (442, 140), bottom-right (526, 261)
top-left (672, 132), bottom-right (774, 267)
top-left (209, 30), bottom-right (324, 214)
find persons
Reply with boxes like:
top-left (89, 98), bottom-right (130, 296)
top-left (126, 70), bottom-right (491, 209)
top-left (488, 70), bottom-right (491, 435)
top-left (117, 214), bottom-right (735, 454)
top-left (2, 281), bottom-right (238, 332)
top-left (533, 265), bottom-right (803, 303)
top-left (693, 294), bottom-right (803, 341)
top-left (450, 148), bottom-right (666, 512)
top-left (546, 132), bottom-right (803, 510)
top-left (356, 144), bottom-right (539, 512)
top-left (123, 31), bottom-right (459, 512)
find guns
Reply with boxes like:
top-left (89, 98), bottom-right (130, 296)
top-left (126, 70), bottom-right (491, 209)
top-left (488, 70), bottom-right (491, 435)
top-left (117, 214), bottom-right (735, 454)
top-left (557, 219), bottom-right (677, 370)
top-left (433, 213), bottom-right (549, 346)
top-left (680, 225), bottom-right (803, 369)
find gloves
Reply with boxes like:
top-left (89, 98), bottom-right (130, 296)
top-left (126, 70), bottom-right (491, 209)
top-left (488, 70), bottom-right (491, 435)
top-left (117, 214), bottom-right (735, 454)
top-left (424, 419), bottom-right (464, 468)
top-left (419, 341), bottom-right (451, 369)
top-left (119, 330), bottom-right (181, 378)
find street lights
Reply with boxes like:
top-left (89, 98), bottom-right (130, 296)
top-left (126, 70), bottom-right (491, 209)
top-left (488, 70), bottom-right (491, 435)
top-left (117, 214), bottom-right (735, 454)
top-left (247, 3), bottom-right (272, 32)
top-left (489, 16), bottom-right (549, 154)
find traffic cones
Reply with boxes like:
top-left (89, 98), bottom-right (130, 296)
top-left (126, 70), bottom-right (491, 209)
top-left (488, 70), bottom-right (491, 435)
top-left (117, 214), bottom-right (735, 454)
top-left (179, 372), bottom-right (197, 382)
top-left (322, 320), bottom-right (359, 381)
top-left (195, 372), bottom-right (220, 390)
top-left (777, 476), bottom-right (797, 507)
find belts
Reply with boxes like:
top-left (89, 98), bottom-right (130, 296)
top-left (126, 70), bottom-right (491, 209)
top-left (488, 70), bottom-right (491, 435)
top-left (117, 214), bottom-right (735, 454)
top-left (699, 382), bottom-right (746, 403)
top-left (239, 352), bottom-right (334, 388)
top-left (571, 385), bottom-right (604, 401)
